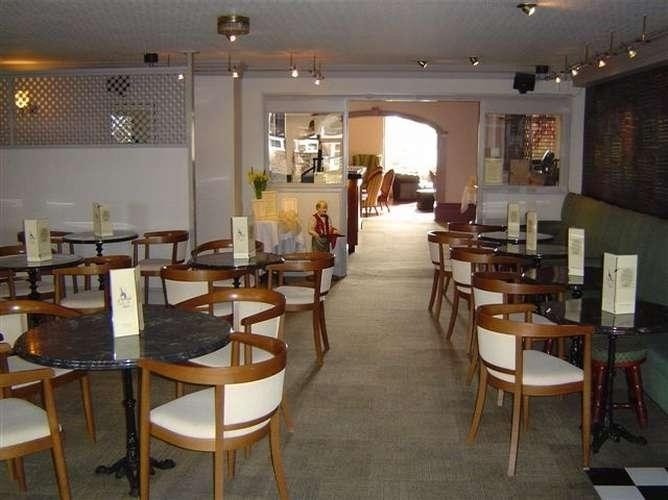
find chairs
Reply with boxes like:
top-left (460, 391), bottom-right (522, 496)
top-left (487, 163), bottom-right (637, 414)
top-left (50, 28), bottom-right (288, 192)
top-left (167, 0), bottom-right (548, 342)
top-left (161, 265), bottom-right (251, 320)
top-left (131, 230), bottom-right (189, 304)
top-left (362, 168), bottom-right (383, 194)
top-left (192, 239), bottom-right (264, 290)
top-left (444, 222), bottom-right (496, 295)
top-left (428, 231), bottom-right (477, 321)
top-left (462, 271), bottom-right (567, 401)
top-left (0, 246), bottom-right (60, 324)
top-left (138, 331), bottom-right (290, 500)
top-left (363, 172), bottom-right (382, 217)
top-left (0, 343), bottom-right (71, 500)
top-left (17, 230), bottom-right (78, 296)
top-left (266, 253), bottom-right (337, 366)
top-left (177, 288), bottom-right (285, 481)
top-left (1, 301), bottom-right (96, 492)
top-left (470, 305), bottom-right (591, 478)
top-left (377, 169), bottom-right (394, 212)
top-left (444, 248), bottom-right (532, 343)
top-left (51, 255), bottom-right (131, 323)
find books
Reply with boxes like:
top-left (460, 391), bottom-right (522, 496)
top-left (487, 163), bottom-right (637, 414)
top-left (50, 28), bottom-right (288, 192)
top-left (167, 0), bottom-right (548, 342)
top-left (22, 218), bottom-right (53, 263)
top-left (90, 201), bottom-right (113, 237)
top-left (601, 252), bottom-right (639, 329)
top-left (110, 266), bottom-right (145, 337)
top-left (506, 202), bottom-right (520, 239)
top-left (566, 228), bottom-right (587, 285)
top-left (230, 216), bottom-right (257, 259)
top-left (525, 210), bottom-right (538, 253)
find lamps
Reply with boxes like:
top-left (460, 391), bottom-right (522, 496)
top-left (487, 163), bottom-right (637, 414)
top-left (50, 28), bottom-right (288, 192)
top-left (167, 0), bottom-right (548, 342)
top-left (217, 15), bottom-right (252, 42)
top-left (554, 57), bottom-right (567, 83)
top-left (598, 32), bottom-right (614, 68)
top-left (628, 16), bottom-right (647, 60)
top-left (571, 45), bottom-right (587, 78)
top-left (227, 55), bottom-right (240, 78)
top-left (286, 54), bottom-right (298, 78)
top-left (311, 58), bottom-right (327, 87)
top-left (469, 57), bottom-right (480, 66)
top-left (516, 4), bottom-right (538, 19)
top-left (416, 59), bottom-right (427, 68)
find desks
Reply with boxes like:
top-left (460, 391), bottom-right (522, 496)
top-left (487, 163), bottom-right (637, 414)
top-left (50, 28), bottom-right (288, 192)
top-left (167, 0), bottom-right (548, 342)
top-left (62, 229), bottom-right (139, 292)
top-left (477, 233), bottom-right (554, 243)
top-left (1, 255), bottom-right (85, 326)
top-left (188, 252), bottom-right (284, 290)
top-left (495, 244), bottom-right (603, 367)
top-left (533, 297), bottom-right (668, 454)
top-left (13, 306), bottom-right (231, 497)
top-left (250, 218), bottom-right (294, 285)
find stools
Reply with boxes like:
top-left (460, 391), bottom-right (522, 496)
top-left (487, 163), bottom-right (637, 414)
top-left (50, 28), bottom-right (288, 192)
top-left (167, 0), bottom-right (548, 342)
top-left (591, 334), bottom-right (640, 432)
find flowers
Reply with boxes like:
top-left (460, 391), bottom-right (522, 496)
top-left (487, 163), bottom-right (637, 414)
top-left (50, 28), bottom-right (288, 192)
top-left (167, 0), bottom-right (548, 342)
top-left (246, 167), bottom-right (271, 198)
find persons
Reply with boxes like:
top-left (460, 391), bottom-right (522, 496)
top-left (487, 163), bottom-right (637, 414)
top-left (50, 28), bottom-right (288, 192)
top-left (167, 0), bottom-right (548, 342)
top-left (307, 201), bottom-right (345, 256)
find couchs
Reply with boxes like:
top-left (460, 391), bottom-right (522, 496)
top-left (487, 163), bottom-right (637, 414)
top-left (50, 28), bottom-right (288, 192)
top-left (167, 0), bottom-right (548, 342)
top-left (518, 193), bottom-right (668, 417)
